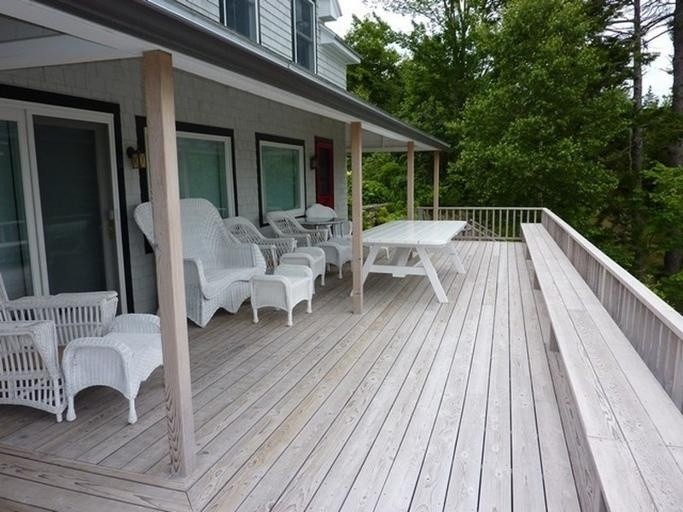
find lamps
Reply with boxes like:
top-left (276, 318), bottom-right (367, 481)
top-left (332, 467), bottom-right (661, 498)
top-left (127, 146), bottom-right (146, 169)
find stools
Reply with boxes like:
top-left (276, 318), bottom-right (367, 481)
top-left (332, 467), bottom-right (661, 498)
top-left (60, 313), bottom-right (163, 426)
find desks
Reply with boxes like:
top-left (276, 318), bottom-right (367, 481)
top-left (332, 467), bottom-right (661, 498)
top-left (357, 219), bottom-right (470, 303)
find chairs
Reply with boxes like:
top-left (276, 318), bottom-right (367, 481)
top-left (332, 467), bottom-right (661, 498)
top-left (133, 197), bottom-right (352, 332)
top-left (0, 275), bottom-right (119, 425)
